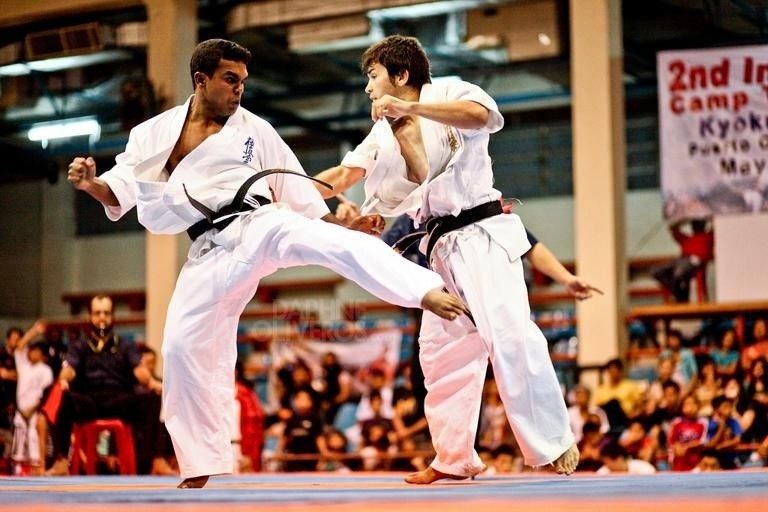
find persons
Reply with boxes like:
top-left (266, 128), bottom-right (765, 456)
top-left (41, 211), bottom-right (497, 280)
top-left (649, 218), bottom-right (713, 303)
top-left (1, 292), bottom-right (767, 475)
top-left (305, 34), bottom-right (579, 485)
top-left (333, 193), bottom-right (605, 469)
top-left (66, 39), bottom-right (467, 491)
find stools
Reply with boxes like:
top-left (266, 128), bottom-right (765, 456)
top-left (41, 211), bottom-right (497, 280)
top-left (70, 419), bottom-right (138, 475)
